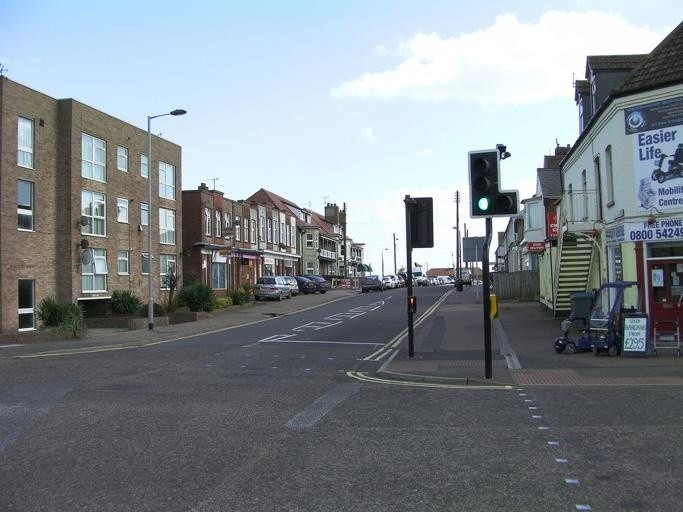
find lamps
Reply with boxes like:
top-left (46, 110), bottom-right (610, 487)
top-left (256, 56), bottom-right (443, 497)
top-left (647, 206), bottom-right (663, 226)
top-left (591, 220), bottom-right (607, 239)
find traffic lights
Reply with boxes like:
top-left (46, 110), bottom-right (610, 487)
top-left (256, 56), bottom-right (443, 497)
top-left (408, 296), bottom-right (416, 313)
top-left (468, 149), bottom-right (520, 218)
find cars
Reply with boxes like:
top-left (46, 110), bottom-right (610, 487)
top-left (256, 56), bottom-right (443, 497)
top-left (382, 272), bottom-right (444, 289)
top-left (253, 275), bottom-right (332, 301)
top-left (360, 275), bottom-right (387, 293)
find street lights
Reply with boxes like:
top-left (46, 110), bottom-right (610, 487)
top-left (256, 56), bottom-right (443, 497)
top-left (148, 109), bottom-right (186, 330)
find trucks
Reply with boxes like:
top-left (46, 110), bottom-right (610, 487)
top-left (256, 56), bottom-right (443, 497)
top-left (437, 275), bottom-right (454, 284)
top-left (455, 270), bottom-right (473, 286)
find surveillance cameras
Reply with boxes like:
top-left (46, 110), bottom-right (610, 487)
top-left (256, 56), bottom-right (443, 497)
top-left (496, 143), bottom-right (506, 153)
